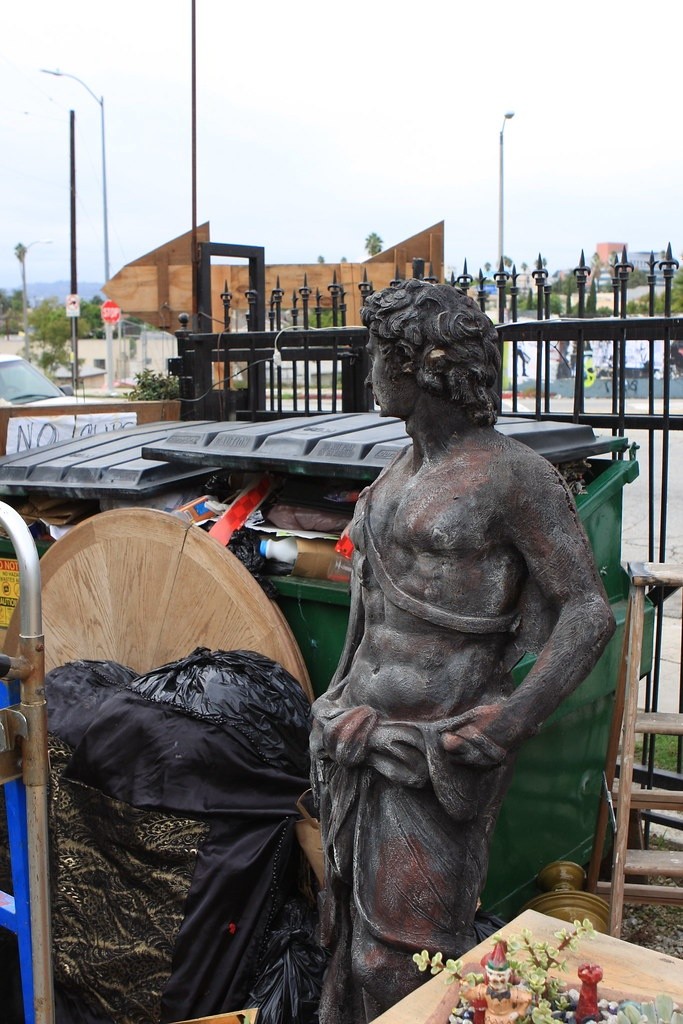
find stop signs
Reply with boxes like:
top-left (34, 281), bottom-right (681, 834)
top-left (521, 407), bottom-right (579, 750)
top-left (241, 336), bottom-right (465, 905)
top-left (100, 300), bottom-right (121, 324)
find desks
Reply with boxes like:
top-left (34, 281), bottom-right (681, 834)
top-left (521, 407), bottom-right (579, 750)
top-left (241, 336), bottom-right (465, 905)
top-left (369, 910), bottom-right (683, 1024)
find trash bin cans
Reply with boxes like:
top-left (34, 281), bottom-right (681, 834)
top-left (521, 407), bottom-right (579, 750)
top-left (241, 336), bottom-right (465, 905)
top-left (0, 407), bottom-right (653, 931)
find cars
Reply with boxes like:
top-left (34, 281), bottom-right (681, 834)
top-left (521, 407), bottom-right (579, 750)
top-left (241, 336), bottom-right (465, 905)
top-left (0, 354), bottom-right (98, 409)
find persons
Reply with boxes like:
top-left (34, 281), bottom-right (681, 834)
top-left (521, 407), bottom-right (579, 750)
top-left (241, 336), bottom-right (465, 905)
top-left (305, 279), bottom-right (615, 1024)
top-left (458, 941), bottom-right (532, 1024)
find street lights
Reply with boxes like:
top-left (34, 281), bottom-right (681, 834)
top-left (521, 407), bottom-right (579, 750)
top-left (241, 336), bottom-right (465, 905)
top-left (496, 112), bottom-right (512, 325)
top-left (41, 68), bottom-right (113, 392)
top-left (21, 240), bottom-right (54, 363)
top-left (118, 320), bottom-right (147, 382)
